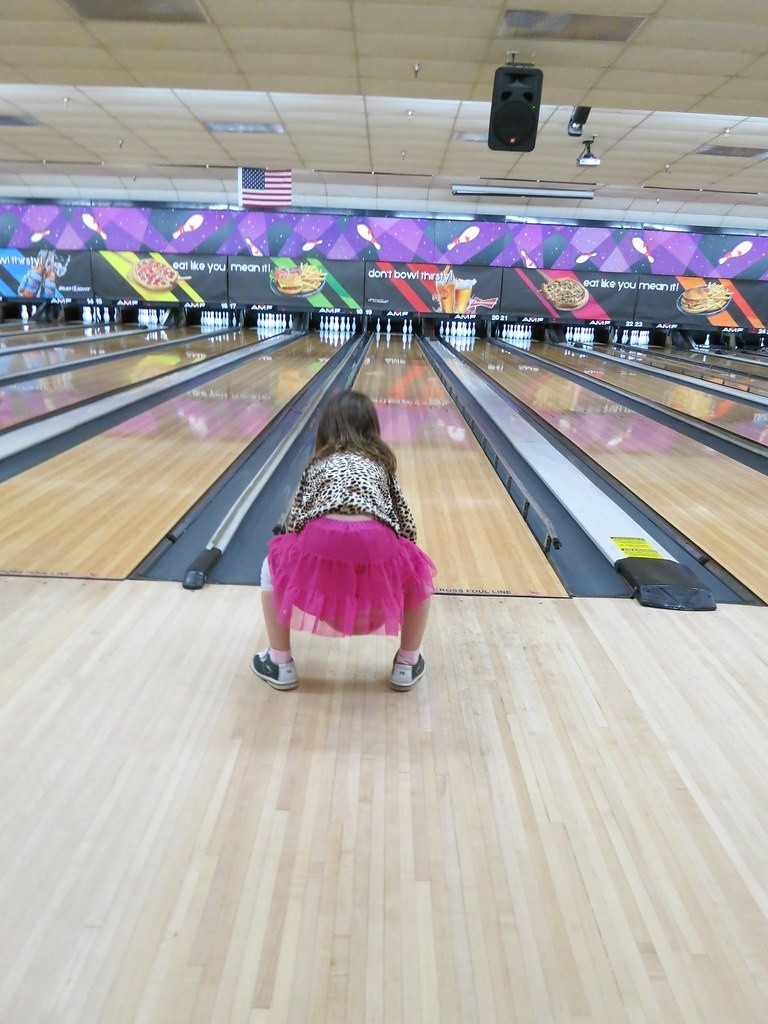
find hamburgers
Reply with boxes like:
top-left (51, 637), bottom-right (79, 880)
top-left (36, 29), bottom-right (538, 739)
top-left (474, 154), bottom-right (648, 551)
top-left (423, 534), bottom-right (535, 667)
top-left (277, 273), bottom-right (303, 295)
top-left (681, 289), bottom-right (708, 313)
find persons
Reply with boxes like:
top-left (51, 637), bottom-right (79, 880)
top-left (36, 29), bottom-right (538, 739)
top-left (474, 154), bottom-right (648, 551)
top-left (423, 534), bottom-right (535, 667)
top-left (250, 389), bottom-right (439, 691)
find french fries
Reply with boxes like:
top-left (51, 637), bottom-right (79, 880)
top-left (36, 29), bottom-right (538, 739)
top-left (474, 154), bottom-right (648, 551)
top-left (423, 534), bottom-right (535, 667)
top-left (299, 263), bottom-right (327, 293)
top-left (706, 282), bottom-right (733, 311)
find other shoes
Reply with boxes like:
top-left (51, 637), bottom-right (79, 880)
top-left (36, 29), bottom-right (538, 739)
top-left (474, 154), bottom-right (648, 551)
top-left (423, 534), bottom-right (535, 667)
top-left (390, 649), bottom-right (426, 691)
top-left (252, 648), bottom-right (298, 690)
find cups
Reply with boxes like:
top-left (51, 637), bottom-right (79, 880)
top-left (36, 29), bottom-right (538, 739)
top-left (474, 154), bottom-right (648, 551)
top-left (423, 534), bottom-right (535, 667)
top-left (436, 283), bottom-right (472, 314)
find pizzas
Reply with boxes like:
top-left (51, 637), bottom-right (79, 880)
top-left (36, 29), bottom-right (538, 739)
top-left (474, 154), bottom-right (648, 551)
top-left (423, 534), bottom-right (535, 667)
top-left (132, 258), bottom-right (179, 292)
top-left (542, 277), bottom-right (588, 308)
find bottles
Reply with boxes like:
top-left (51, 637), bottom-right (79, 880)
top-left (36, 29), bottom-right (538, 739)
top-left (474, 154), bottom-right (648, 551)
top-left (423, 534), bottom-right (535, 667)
top-left (21, 249), bottom-right (55, 298)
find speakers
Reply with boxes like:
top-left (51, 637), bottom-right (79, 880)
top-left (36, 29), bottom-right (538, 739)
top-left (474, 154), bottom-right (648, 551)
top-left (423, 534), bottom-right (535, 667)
top-left (488, 66), bottom-right (543, 151)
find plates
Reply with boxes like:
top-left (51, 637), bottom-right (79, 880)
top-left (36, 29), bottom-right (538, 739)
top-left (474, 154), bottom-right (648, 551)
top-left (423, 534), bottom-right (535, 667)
top-left (554, 288), bottom-right (589, 311)
top-left (676, 286), bottom-right (731, 316)
top-left (270, 267), bottom-right (325, 298)
top-left (133, 259), bottom-right (178, 292)
